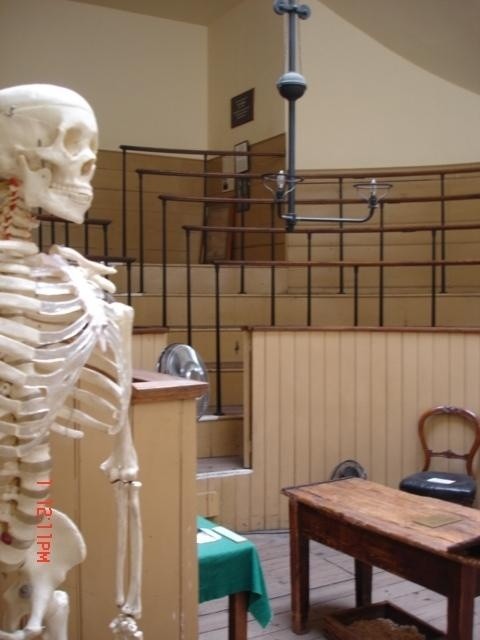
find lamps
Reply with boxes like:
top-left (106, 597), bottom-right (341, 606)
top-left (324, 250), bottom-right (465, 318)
top-left (260, 168), bottom-right (393, 222)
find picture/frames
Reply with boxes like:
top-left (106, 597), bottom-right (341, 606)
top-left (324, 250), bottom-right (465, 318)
top-left (199, 202), bottom-right (235, 262)
top-left (233, 140), bottom-right (249, 173)
top-left (230, 88), bottom-right (254, 128)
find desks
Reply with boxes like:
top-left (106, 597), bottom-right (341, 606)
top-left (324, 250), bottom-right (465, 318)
top-left (282, 476), bottom-right (480, 640)
top-left (197, 515), bottom-right (272, 640)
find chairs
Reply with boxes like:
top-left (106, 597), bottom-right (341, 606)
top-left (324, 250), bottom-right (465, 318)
top-left (398, 406), bottom-right (480, 507)
